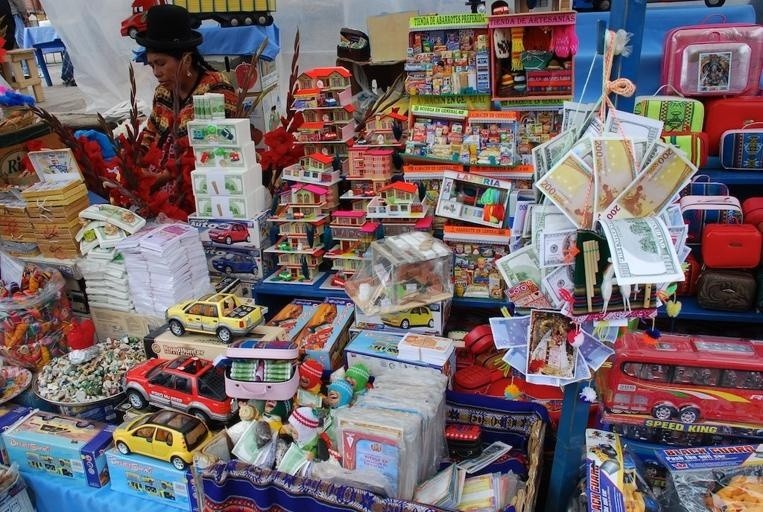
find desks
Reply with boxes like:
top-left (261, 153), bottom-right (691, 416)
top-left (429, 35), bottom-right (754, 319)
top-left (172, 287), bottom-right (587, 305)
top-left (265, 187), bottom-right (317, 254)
top-left (25, 26), bottom-right (73, 86)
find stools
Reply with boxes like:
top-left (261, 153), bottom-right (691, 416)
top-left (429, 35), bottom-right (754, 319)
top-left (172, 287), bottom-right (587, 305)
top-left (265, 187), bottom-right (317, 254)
top-left (2, 49), bottom-right (44, 105)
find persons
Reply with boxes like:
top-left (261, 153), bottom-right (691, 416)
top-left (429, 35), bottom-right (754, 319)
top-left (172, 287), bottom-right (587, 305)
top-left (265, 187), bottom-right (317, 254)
top-left (0, 1), bottom-right (29, 81)
top-left (133, 5), bottom-right (263, 197)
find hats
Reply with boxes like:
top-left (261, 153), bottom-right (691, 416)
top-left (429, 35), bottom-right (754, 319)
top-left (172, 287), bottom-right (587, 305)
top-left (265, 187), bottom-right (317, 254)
top-left (136, 5), bottom-right (202, 48)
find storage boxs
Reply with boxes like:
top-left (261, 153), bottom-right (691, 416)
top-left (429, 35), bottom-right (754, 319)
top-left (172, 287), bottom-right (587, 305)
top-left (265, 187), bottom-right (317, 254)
top-left (0, 277), bottom-right (549, 510)
top-left (192, 26), bottom-right (286, 154)
